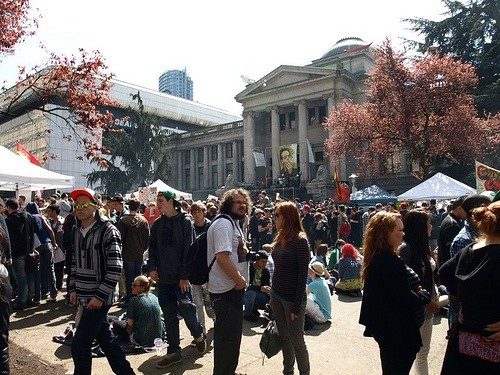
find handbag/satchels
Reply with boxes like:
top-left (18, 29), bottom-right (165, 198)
top-left (459, 331), bottom-right (500, 361)
top-left (259, 316), bottom-right (282, 366)
top-left (340, 221), bottom-right (350, 237)
top-left (52, 245), bottom-right (65, 263)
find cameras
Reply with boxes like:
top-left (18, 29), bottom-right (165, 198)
top-left (246, 251), bottom-right (260, 262)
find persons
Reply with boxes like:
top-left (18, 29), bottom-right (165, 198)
top-left (358, 211), bottom-right (438, 375)
top-left (206, 187), bottom-right (251, 375)
top-left (279, 148), bottom-right (299, 186)
top-left (0, 187), bottom-right (500, 375)
top-left (268, 200), bottom-right (310, 375)
top-left (398, 208), bottom-right (440, 375)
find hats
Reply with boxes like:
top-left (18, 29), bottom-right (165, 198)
top-left (255, 209), bottom-right (264, 214)
top-left (113, 196), bottom-right (123, 201)
top-left (4, 200), bottom-right (18, 208)
top-left (106, 196), bottom-right (113, 201)
top-left (26, 203), bottom-right (39, 214)
top-left (70, 188), bottom-right (97, 203)
top-left (157, 191), bottom-right (188, 209)
top-left (256, 250), bottom-right (268, 259)
top-left (308, 262), bottom-right (325, 276)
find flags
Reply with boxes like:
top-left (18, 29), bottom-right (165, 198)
top-left (474, 159), bottom-right (500, 195)
top-left (16, 140), bottom-right (41, 167)
top-left (332, 164), bottom-right (350, 202)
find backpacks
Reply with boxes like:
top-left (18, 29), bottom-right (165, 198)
top-left (185, 214), bottom-right (235, 285)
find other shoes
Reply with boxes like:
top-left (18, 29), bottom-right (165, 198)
top-left (191, 335), bottom-right (207, 346)
top-left (243, 311), bottom-right (260, 321)
top-left (346, 290), bottom-right (362, 298)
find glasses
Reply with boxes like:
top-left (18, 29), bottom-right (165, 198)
top-left (131, 283), bottom-right (143, 287)
top-left (232, 201), bottom-right (247, 205)
top-left (75, 202), bottom-right (95, 209)
top-left (274, 213), bottom-right (280, 218)
top-left (39, 203), bottom-right (42, 204)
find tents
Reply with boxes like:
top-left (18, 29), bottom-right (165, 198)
top-left (0, 145), bottom-right (76, 200)
top-left (397, 172), bottom-right (477, 202)
top-left (123, 178), bottom-right (193, 205)
top-left (335, 184), bottom-right (397, 207)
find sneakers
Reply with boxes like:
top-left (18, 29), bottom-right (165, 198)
top-left (194, 333), bottom-right (206, 353)
top-left (157, 348), bottom-right (182, 369)
